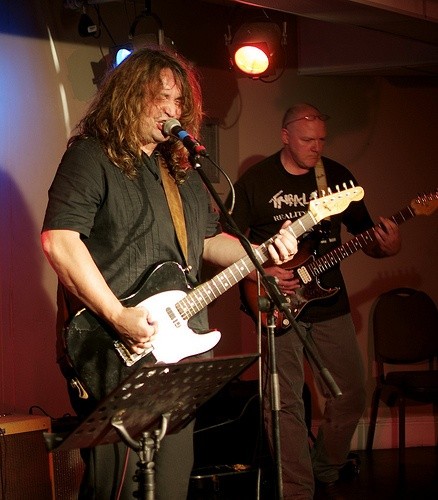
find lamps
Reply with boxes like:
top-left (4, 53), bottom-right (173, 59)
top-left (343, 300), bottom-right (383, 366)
top-left (222, 15), bottom-right (290, 82)
top-left (110, 0), bottom-right (187, 69)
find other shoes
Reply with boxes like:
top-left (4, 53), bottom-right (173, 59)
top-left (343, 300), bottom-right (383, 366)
top-left (316, 480), bottom-right (338, 500)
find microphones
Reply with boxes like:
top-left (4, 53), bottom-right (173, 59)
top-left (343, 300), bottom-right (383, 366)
top-left (162, 118), bottom-right (209, 158)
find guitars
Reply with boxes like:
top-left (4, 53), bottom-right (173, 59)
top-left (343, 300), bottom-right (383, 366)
top-left (240, 191), bottom-right (438, 331)
top-left (59, 180), bottom-right (366, 408)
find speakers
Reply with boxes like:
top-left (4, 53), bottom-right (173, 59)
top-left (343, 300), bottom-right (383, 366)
top-left (0, 413), bottom-right (87, 500)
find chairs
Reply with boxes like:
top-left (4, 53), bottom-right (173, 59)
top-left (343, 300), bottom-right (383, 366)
top-left (366, 287), bottom-right (438, 474)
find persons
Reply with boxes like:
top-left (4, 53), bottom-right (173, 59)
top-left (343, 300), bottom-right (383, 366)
top-left (40, 48), bottom-right (297, 500)
top-left (219, 105), bottom-right (400, 500)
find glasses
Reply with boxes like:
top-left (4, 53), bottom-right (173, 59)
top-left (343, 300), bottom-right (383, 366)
top-left (283, 113), bottom-right (330, 128)
